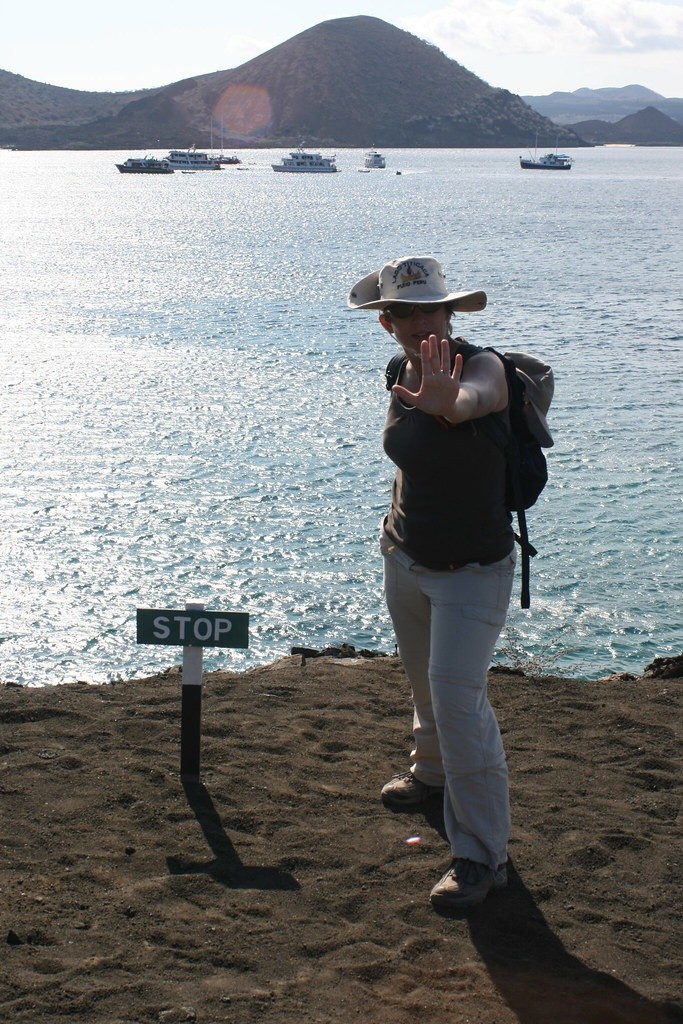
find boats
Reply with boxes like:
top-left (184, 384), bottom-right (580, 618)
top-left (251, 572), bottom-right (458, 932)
top-left (270, 152), bottom-right (341, 172)
top-left (211, 155), bottom-right (242, 165)
top-left (181, 170), bottom-right (196, 175)
top-left (358, 168), bottom-right (370, 173)
top-left (114, 155), bottom-right (175, 174)
top-left (236, 167), bottom-right (248, 170)
top-left (161, 144), bottom-right (223, 170)
top-left (364, 149), bottom-right (386, 169)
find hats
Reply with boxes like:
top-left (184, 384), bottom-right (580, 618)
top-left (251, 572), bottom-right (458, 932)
top-left (347, 255), bottom-right (487, 312)
top-left (503, 351), bottom-right (554, 449)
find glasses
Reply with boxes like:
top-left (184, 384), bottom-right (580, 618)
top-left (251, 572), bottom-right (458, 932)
top-left (383, 303), bottom-right (445, 317)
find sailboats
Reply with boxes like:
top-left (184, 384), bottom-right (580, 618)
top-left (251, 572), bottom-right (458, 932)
top-left (519, 132), bottom-right (573, 170)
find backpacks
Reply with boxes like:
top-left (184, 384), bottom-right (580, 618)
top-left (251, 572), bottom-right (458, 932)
top-left (386, 341), bottom-right (547, 512)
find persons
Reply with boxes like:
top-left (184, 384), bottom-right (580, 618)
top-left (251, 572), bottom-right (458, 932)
top-left (348, 254), bottom-right (517, 913)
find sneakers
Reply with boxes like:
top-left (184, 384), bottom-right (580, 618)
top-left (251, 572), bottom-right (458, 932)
top-left (380, 770), bottom-right (444, 805)
top-left (430, 858), bottom-right (513, 909)
